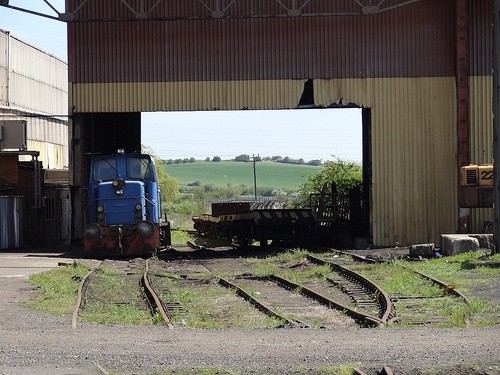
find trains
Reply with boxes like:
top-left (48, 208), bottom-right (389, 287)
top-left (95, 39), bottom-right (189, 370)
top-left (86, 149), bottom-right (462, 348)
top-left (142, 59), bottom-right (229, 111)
top-left (84, 148), bottom-right (161, 256)
top-left (192, 202), bottom-right (317, 250)
